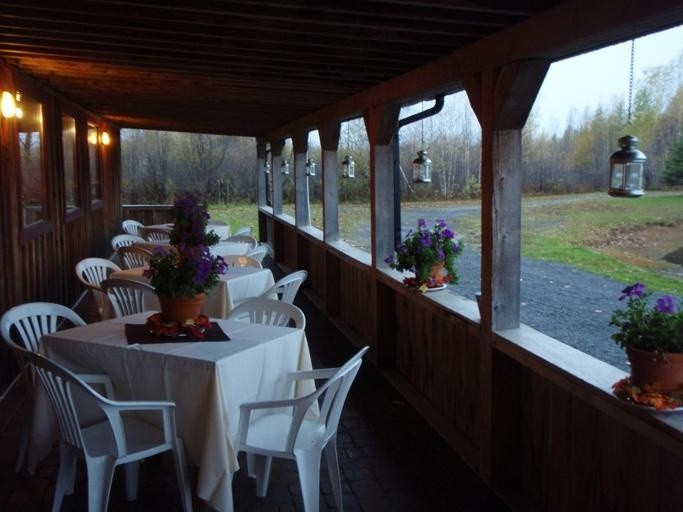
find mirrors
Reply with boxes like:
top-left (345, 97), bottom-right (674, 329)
top-left (11, 80), bottom-right (55, 247)
top-left (83, 112), bottom-right (106, 213)
top-left (58, 105), bottom-right (85, 222)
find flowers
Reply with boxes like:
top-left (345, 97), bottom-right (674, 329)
top-left (609, 283), bottom-right (683, 353)
top-left (384, 218), bottom-right (465, 290)
top-left (142, 245), bottom-right (228, 298)
top-left (168, 221), bottom-right (220, 247)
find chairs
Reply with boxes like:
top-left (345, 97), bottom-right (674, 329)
top-left (121, 219), bottom-right (143, 235)
top-left (234, 226), bottom-right (250, 235)
top-left (1, 302), bottom-right (88, 495)
top-left (111, 233), bottom-right (145, 251)
top-left (234, 345), bottom-right (371, 512)
top-left (245, 246), bottom-right (268, 265)
top-left (22, 348), bottom-right (192, 512)
top-left (139, 228), bottom-right (170, 241)
top-left (220, 253), bottom-right (261, 268)
top-left (264, 269), bottom-right (309, 326)
top-left (118, 245), bottom-right (152, 269)
top-left (225, 235), bottom-right (257, 248)
top-left (75, 257), bottom-right (120, 315)
top-left (225, 298), bottom-right (307, 332)
top-left (101, 277), bottom-right (155, 315)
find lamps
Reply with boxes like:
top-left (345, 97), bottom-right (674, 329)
top-left (305, 132), bottom-right (316, 176)
top-left (280, 159), bottom-right (289, 175)
top-left (607, 39), bottom-right (647, 198)
top-left (263, 161), bottom-right (270, 174)
top-left (341, 122), bottom-right (355, 179)
top-left (412, 99), bottom-right (433, 184)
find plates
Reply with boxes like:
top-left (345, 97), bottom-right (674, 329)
top-left (611, 386), bottom-right (682, 412)
top-left (412, 283), bottom-right (448, 291)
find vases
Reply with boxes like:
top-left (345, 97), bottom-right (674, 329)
top-left (415, 262), bottom-right (444, 279)
top-left (173, 244), bottom-right (201, 259)
top-left (624, 347), bottom-right (683, 395)
top-left (156, 293), bottom-right (204, 321)
